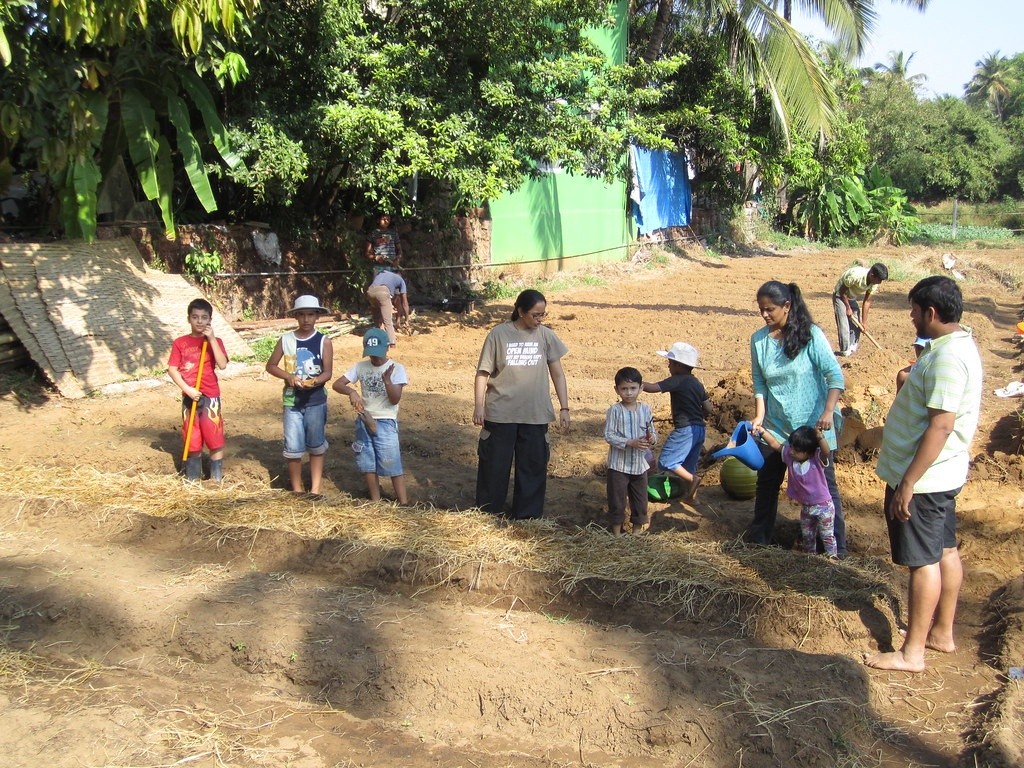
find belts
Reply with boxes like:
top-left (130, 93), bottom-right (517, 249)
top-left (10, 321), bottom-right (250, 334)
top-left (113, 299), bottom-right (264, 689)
top-left (378, 284), bottom-right (386, 287)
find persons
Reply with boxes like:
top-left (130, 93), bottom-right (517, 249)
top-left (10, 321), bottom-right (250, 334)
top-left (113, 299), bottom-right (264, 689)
top-left (897, 333), bottom-right (932, 396)
top-left (472, 290), bottom-right (572, 521)
top-left (832, 263), bottom-right (889, 358)
top-left (604, 367), bottom-right (657, 539)
top-left (857, 275), bottom-right (982, 666)
top-left (747, 420), bottom-right (838, 561)
top-left (332, 328), bottom-right (409, 507)
top-left (365, 270), bottom-right (410, 349)
top-left (641, 343), bottom-right (713, 504)
top-left (168, 299), bottom-right (228, 484)
top-left (727, 281), bottom-right (851, 560)
top-left (264, 295), bottom-right (333, 502)
top-left (365, 211), bottom-right (406, 333)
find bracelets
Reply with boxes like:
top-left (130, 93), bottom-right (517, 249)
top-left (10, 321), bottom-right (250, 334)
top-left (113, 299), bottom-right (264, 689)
top-left (560, 408), bottom-right (570, 411)
top-left (816, 434), bottom-right (825, 442)
top-left (759, 428), bottom-right (767, 436)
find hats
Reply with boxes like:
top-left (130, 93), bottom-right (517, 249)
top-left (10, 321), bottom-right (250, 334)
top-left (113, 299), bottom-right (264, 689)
top-left (913, 334), bottom-right (932, 348)
top-left (656, 342), bottom-right (703, 369)
top-left (285, 295), bottom-right (331, 318)
top-left (362, 327), bottom-right (391, 358)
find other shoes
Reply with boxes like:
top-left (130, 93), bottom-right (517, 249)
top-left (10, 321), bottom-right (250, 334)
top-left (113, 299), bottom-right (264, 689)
top-left (390, 343), bottom-right (396, 348)
top-left (850, 343), bottom-right (857, 352)
top-left (840, 349), bottom-right (851, 357)
top-left (687, 474), bottom-right (702, 499)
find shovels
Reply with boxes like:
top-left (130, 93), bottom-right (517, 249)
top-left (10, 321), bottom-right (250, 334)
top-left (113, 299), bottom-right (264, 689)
top-left (175, 322), bottom-right (211, 478)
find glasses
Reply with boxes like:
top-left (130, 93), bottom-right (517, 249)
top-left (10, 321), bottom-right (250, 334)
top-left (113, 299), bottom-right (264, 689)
top-left (527, 311), bottom-right (548, 320)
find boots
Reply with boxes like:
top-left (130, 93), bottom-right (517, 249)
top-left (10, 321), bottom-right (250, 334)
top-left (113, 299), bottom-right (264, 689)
top-left (186, 456), bottom-right (203, 481)
top-left (210, 458), bottom-right (222, 483)
